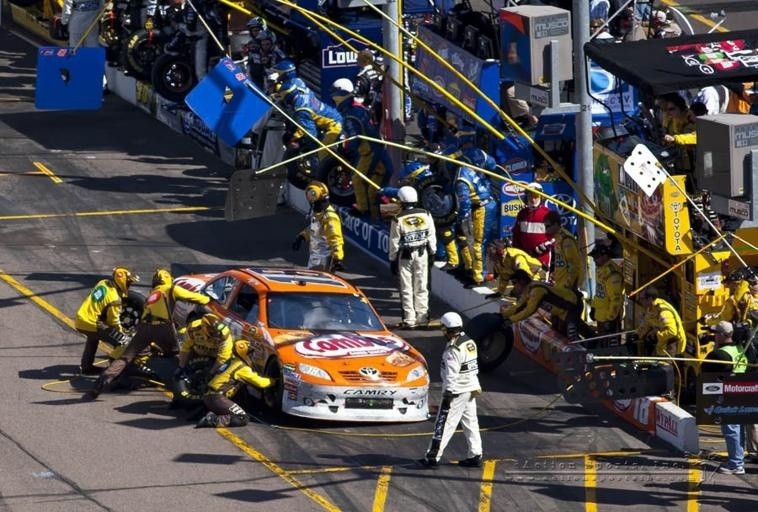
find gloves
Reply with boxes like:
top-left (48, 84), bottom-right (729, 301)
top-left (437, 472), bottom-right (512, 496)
top-left (292, 235), bottom-right (304, 250)
top-left (428, 254), bottom-right (435, 266)
top-left (391, 260), bottom-right (399, 276)
top-left (441, 390), bottom-right (453, 409)
top-left (333, 258), bottom-right (343, 267)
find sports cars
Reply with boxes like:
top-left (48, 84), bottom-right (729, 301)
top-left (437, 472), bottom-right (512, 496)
top-left (164, 263), bottom-right (433, 424)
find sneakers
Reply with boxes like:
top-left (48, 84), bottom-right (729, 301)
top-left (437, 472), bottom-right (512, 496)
top-left (198, 411), bottom-right (217, 428)
top-left (719, 466), bottom-right (745, 475)
top-left (459, 455), bottom-right (482, 467)
top-left (412, 458), bottom-right (439, 468)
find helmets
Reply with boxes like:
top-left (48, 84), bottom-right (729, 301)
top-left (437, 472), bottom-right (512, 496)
top-left (248, 16), bottom-right (299, 104)
top-left (114, 267), bottom-right (140, 294)
top-left (233, 339), bottom-right (254, 366)
top-left (305, 181), bottom-right (329, 202)
top-left (401, 162), bottom-right (428, 181)
top-left (525, 181), bottom-right (543, 196)
top-left (440, 311), bottom-right (463, 329)
top-left (442, 125), bottom-right (476, 168)
top-left (330, 77), bottom-right (354, 111)
top-left (185, 318), bottom-right (210, 342)
top-left (152, 269), bottom-right (171, 285)
top-left (398, 186), bottom-right (418, 203)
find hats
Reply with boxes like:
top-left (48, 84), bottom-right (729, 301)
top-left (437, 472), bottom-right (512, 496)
top-left (709, 320), bottom-right (733, 337)
top-left (587, 244), bottom-right (608, 257)
